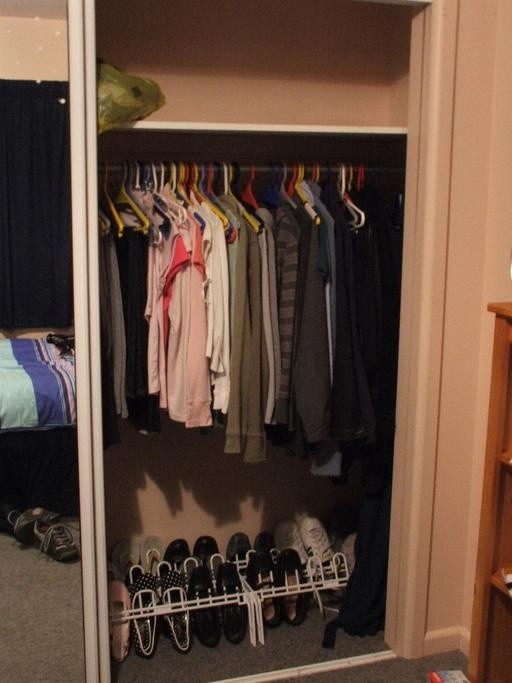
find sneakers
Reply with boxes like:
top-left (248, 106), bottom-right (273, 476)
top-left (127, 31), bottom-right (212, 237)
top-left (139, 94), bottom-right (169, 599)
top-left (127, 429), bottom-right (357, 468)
top-left (6, 508), bottom-right (80, 561)
top-left (108, 516), bottom-right (336, 664)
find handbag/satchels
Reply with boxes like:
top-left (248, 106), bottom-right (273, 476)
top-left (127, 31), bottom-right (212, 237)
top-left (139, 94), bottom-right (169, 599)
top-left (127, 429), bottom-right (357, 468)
top-left (323, 488), bottom-right (391, 648)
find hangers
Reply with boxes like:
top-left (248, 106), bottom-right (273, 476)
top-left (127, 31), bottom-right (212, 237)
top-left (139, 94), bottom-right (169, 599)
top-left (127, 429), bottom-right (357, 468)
top-left (98, 157), bottom-right (367, 246)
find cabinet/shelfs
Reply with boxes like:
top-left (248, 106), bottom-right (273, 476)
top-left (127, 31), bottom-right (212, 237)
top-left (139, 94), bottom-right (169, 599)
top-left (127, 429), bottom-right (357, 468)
top-left (465, 301), bottom-right (512, 683)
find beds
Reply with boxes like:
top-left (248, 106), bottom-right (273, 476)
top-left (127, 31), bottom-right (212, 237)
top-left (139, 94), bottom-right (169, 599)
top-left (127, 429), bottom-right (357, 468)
top-left (0, 337), bottom-right (77, 434)
